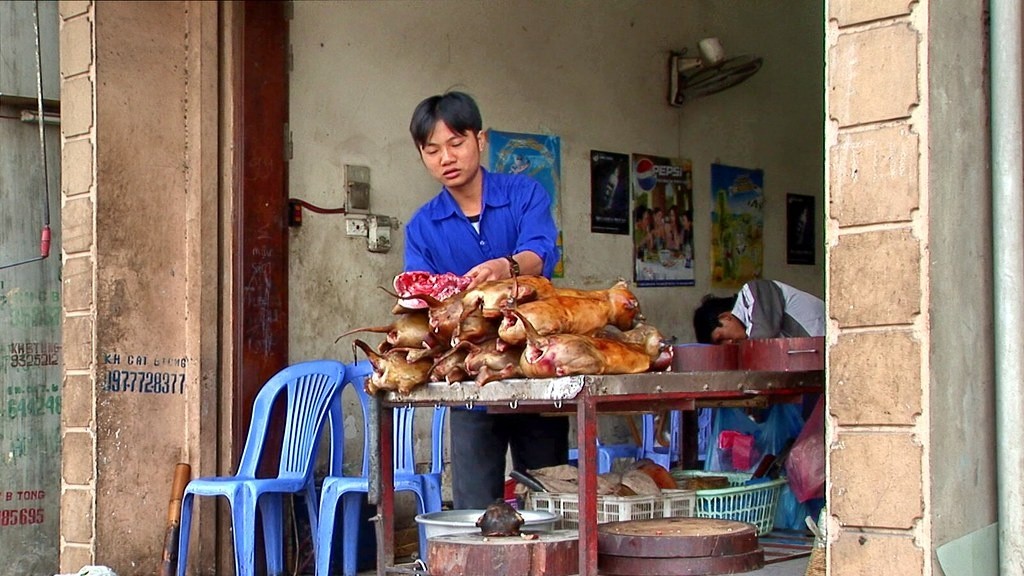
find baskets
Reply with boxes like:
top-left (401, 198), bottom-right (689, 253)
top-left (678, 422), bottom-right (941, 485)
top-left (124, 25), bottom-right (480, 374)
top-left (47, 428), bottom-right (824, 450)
top-left (529, 488), bottom-right (695, 528)
top-left (671, 470), bottom-right (788, 535)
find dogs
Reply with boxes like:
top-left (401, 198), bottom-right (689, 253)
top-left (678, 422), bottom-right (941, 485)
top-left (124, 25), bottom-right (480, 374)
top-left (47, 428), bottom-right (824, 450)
top-left (334, 271), bottom-right (674, 397)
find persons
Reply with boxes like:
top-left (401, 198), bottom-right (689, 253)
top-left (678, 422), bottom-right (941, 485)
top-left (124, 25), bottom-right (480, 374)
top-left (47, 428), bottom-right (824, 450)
top-left (403, 91), bottom-right (572, 511)
top-left (694, 279), bottom-right (826, 420)
top-left (633, 204), bottom-right (695, 268)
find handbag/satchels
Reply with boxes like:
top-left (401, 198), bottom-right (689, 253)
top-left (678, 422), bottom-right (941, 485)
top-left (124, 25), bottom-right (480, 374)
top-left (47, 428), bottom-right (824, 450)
top-left (705, 408), bottom-right (809, 529)
top-left (785, 394), bottom-right (826, 503)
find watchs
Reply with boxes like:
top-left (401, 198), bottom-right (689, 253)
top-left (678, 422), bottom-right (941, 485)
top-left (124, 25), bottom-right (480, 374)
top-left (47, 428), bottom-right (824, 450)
top-left (505, 254), bottom-right (520, 278)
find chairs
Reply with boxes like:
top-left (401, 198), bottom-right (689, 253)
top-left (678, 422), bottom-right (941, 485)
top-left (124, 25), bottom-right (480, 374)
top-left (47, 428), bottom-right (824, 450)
top-left (176, 359), bottom-right (350, 576)
top-left (314, 361), bottom-right (452, 576)
top-left (565, 341), bottom-right (719, 473)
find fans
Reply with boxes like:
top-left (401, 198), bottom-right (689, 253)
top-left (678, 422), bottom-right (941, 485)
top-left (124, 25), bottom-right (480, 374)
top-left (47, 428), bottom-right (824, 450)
top-left (670, 36), bottom-right (765, 106)
